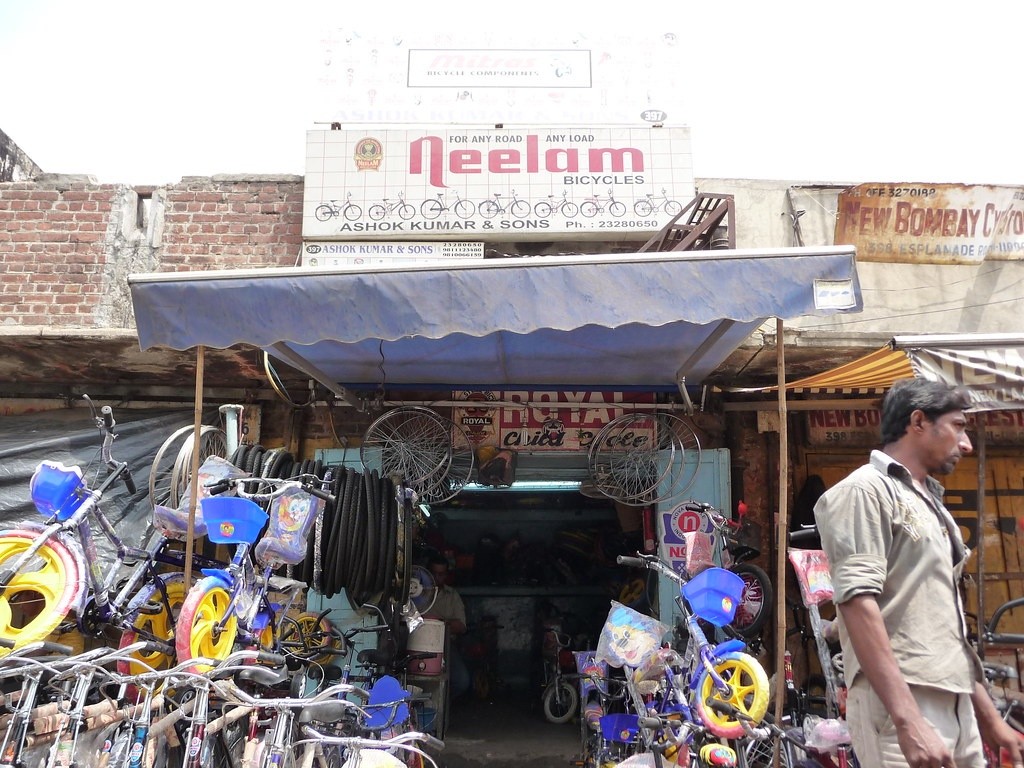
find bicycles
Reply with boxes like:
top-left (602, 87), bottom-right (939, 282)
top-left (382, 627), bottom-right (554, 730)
top-left (0, 393), bottom-right (1023, 768)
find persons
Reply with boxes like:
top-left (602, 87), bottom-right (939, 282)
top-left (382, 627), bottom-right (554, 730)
top-left (813, 376), bottom-right (1024, 768)
top-left (417, 556), bottom-right (466, 705)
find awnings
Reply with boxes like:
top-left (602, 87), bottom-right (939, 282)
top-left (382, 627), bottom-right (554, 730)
top-left (129, 248), bottom-right (863, 767)
top-left (721, 333), bottom-right (1024, 413)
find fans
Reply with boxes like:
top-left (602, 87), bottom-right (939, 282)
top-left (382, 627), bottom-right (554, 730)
top-left (410, 564), bottom-right (438, 615)
